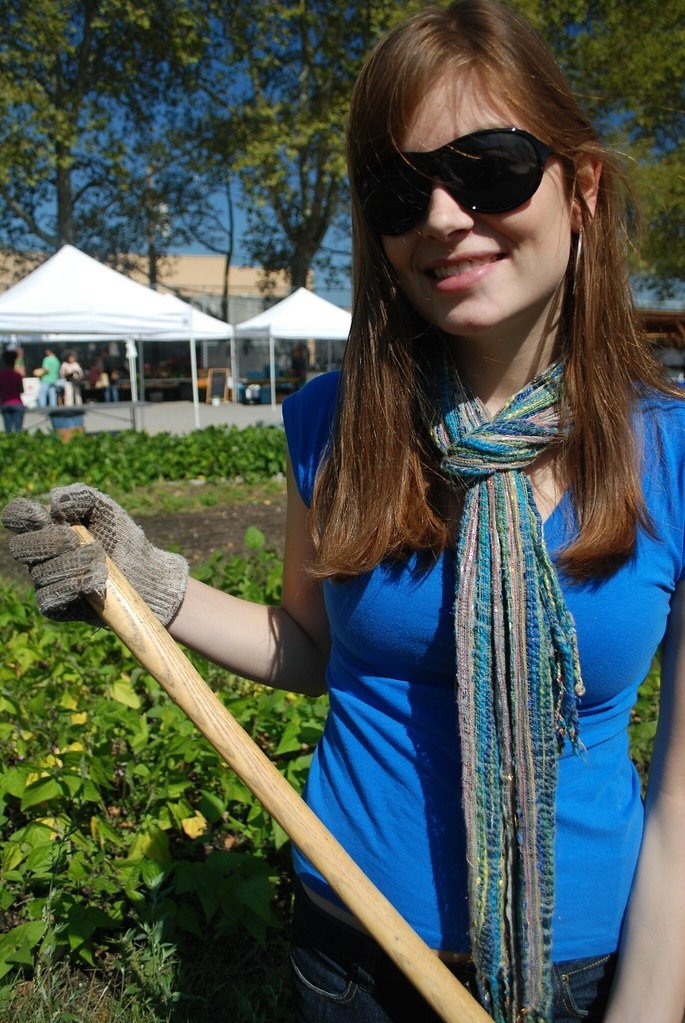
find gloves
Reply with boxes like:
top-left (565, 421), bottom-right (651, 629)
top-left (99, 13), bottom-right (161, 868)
top-left (2, 484), bottom-right (190, 631)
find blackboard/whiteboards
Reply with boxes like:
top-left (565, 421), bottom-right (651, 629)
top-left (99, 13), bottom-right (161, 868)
top-left (208, 368), bottom-right (229, 400)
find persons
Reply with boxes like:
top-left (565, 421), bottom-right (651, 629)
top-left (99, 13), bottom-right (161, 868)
top-left (135, 351), bottom-right (181, 401)
top-left (0, 0), bottom-right (684, 1023)
top-left (289, 344), bottom-right (306, 390)
top-left (59, 348), bottom-right (120, 407)
top-left (0, 351), bottom-right (24, 433)
top-left (33, 346), bottom-right (60, 414)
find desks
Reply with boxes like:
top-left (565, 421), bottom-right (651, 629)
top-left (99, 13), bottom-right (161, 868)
top-left (77, 378), bottom-right (178, 389)
top-left (243, 377), bottom-right (303, 386)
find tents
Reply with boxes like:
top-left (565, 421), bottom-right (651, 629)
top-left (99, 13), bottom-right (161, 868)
top-left (0, 240), bottom-right (235, 432)
top-left (230, 286), bottom-right (352, 407)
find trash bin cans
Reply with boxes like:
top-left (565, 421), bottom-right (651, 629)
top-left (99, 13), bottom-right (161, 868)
top-left (49, 409), bottom-right (86, 439)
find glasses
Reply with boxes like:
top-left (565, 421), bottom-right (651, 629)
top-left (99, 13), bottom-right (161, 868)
top-left (365, 128), bottom-right (576, 237)
